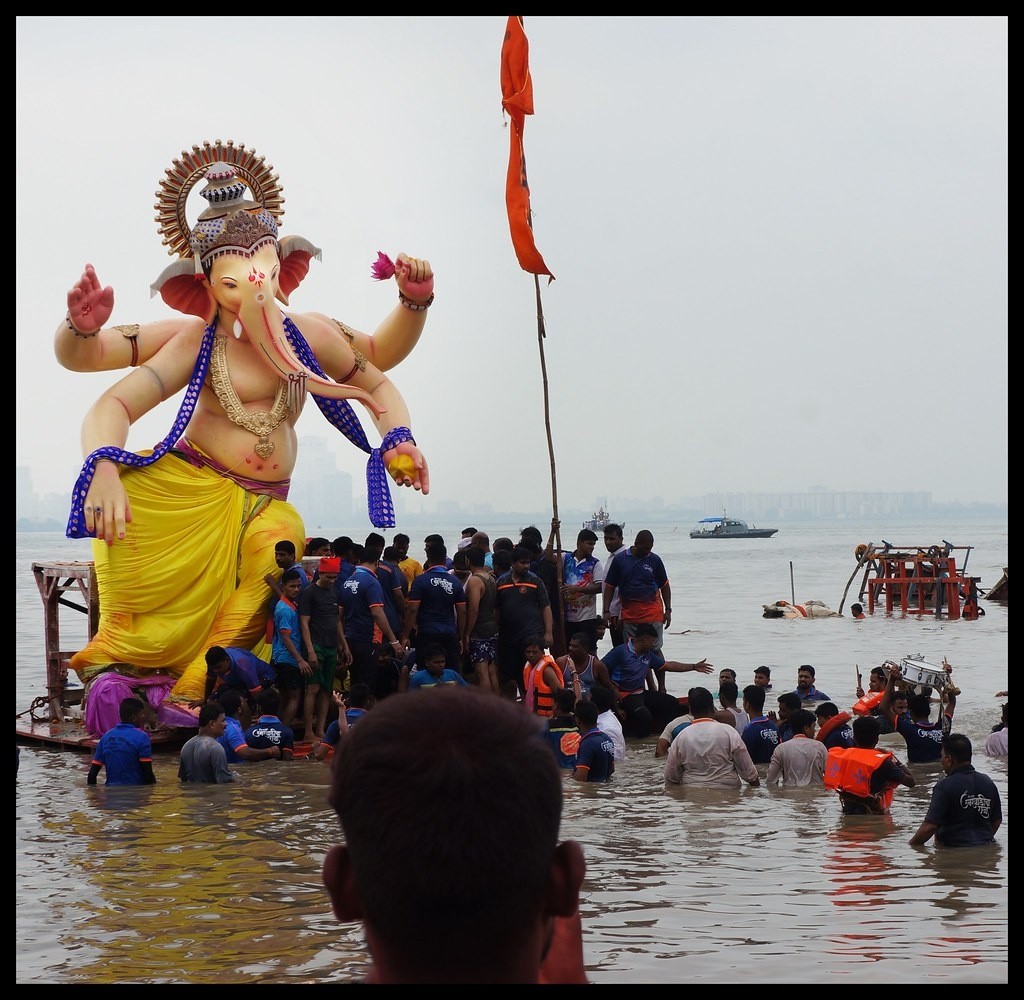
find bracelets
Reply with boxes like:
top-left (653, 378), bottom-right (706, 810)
top-left (65, 310), bottom-right (102, 340)
top-left (397, 290), bottom-right (434, 306)
top-left (693, 664), bottom-right (695, 670)
top-left (398, 294), bottom-right (433, 310)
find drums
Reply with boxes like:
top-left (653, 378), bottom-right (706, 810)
top-left (899, 658), bottom-right (947, 688)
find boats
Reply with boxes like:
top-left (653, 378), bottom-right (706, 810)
top-left (691, 509), bottom-right (778, 538)
top-left (583, 497), bottom-right (626, 532)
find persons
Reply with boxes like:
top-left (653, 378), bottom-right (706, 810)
top-left (851, 603), bottom-right (866, 619)
top-left (876, 663), bottom-right (957, 765)
top-left (824, 717), bottom-right (917, 816)
top-left (204, 523), bottom-right (831, 794)
top-left (175, 702), bottom-right (242, 783)
top-left (767, 709), bottom-right (828, 786)
top-left (985, 701), bottom-right (1008, 756)
top-left (54, 139), bottom-right (434, 736)
top-left (85, 697), bottom-right (157, 786)
top-left (876, 691), bottom-right (908, 734)
top-left (855, 665), bottom-right (888, 715)
top-left (907, 733), bottom-right (1003, 853)
top-left (318, 684), bottom-right (594, 988)
top-left (813, 702), bottom-right (856, 749)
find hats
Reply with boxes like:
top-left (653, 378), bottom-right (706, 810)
top-left (318, 558), bottom-right (341, 574)
top-left (458, 536), bottom-right (474, 550)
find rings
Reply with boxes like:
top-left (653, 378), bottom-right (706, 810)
top-left (93, 506), bottom-right (104, 513)
top-left (408, 256), bottom-right (416, 263)
top-left (84, 506), bottom-right (94, 512)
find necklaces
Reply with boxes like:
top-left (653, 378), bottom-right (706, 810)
top-left (207, 332), bottom-right (293, 461)
